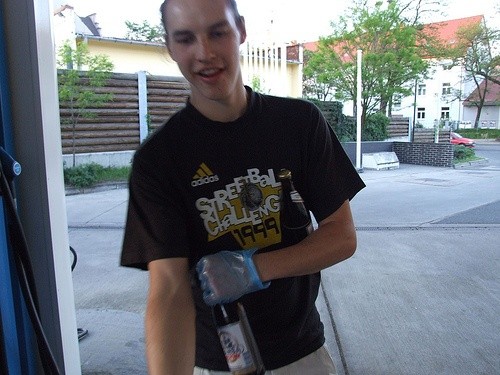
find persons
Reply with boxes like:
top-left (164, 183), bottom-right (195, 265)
top-left (119, 0), bottom-right (366, 375)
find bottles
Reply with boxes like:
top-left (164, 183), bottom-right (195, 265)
top-left (211, 300), bottom-right (266, 375)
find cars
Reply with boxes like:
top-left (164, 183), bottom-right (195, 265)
top-left (449, 130), bottom-right (475, 151)
